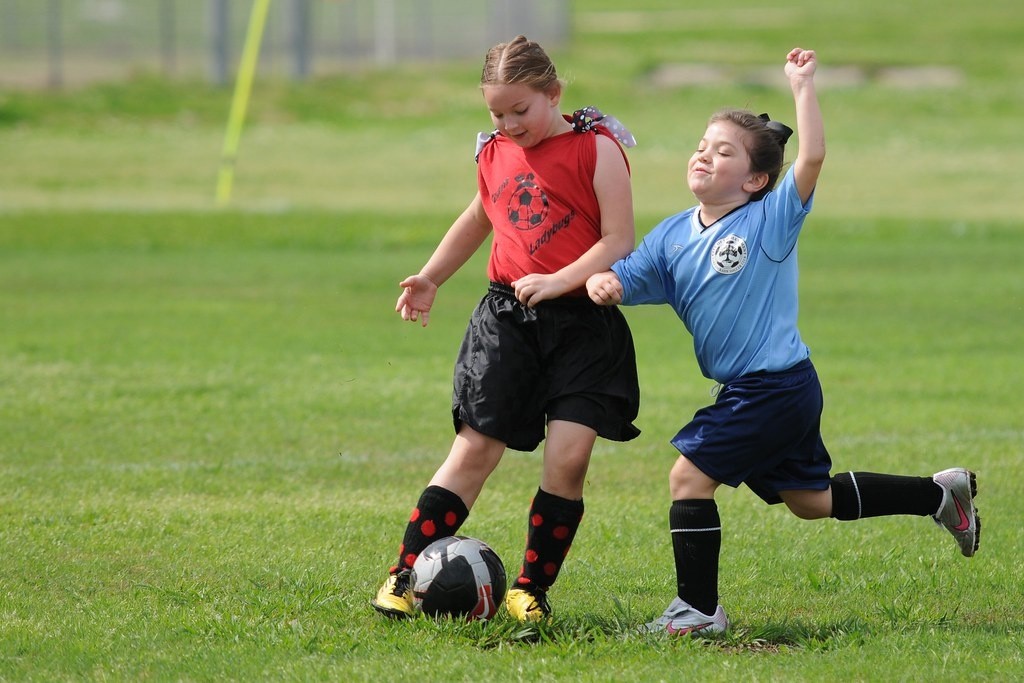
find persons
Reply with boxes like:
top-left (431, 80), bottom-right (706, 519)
top-left (371, 35), bottom-right (642, 633)
top-left (585, 47), bottom-right (982, 648)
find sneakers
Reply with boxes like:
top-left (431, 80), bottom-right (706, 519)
top-left (370, 567), bottom-right (419, 621)
top-left (929, 467), bottom-right (982, 557)
top-left (624, 597), bottom-right (728, 640)
top-left (505, 576), bottom-right (551, 629)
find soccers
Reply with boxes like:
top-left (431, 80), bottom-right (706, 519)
top-left (408, 536), bottom-right (508, 626)
